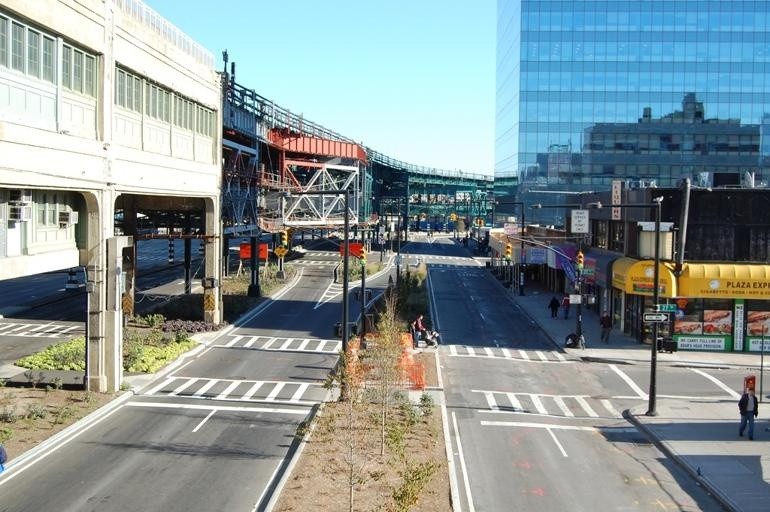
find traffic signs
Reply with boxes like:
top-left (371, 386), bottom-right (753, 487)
top-left (653, 303), bottom-right (677, 312)
top-left (643, 312), bottom-right (669, 324)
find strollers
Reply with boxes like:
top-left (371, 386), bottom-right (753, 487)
top-left (423, 328), bottom-right (440, 351)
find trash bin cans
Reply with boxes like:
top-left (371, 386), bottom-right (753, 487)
top-left (486, 262), bottom-right (490, 269)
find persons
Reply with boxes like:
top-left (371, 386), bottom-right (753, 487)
top-left (408, 320), bottom-right (417, 350)
top-left (738, 387), bottom-right (759, 440)
top-left (546, 295), bottom-right (560, 320)
top-left (415, 314), bottom-right (426, 347)
top-left (560, 293), bottom-right (570, 320)
top-left (424, 328), bottom-right (434, 346)
top-left (598, 311), bottom-right (614, 343)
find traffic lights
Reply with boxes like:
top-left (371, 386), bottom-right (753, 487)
top-left (281, 231), bottom-right (288, 248)
top-left (506, 244), bottom-right (512, 262)
top-left (577, 253), bottom-right (584, 271)
top-left (359, 247), bottom-right (367, 266)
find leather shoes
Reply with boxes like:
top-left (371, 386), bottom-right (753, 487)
top-left (738, 429), bottom-right (754, 441)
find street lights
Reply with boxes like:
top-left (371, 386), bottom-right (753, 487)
top-left (446, 198), bottom-right (482, 254)
top-left (585, 199), bottom-right (664, 419)
top-left (529, 202), bottom-right (585, 345)
top-left (488, 201), bottom-right (527, 298)
top-left (274, 188), bottom-right (351, 403)
